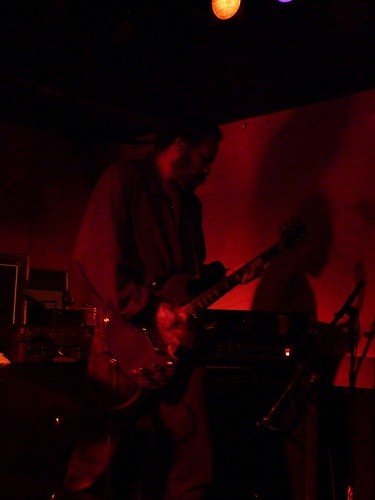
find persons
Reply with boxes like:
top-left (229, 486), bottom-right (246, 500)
top-left (65, 107), bottom-right (229, 499)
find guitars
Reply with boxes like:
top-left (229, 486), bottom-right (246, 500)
top-left (86, 220), bottom-right (307, 413)
